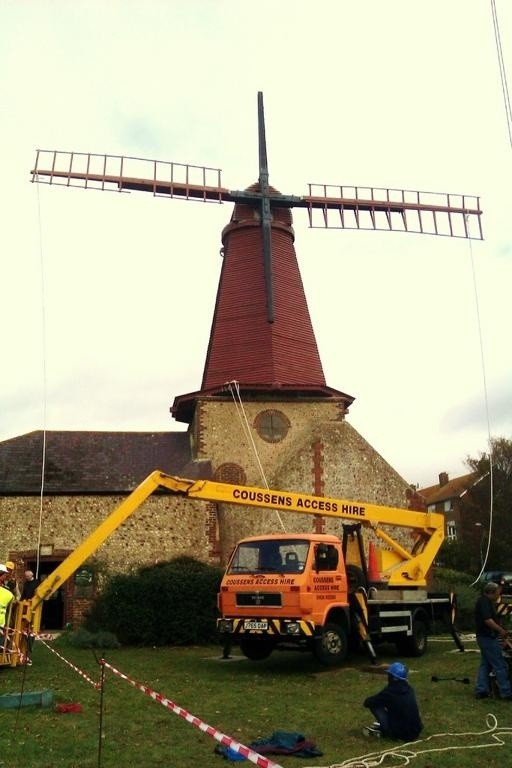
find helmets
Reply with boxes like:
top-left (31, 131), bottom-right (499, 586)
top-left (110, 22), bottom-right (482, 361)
top-left (384, 662), bottom-right (407, 680)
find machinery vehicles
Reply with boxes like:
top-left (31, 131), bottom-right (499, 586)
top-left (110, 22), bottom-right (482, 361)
top-left (3, 468), bottom-right (459, 665)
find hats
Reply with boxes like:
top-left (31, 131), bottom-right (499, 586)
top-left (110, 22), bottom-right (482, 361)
top-left (0, 562), bottom-right (15, 572)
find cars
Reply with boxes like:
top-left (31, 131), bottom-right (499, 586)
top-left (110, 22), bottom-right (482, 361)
top-left (475, 570), bottom-right (512, 593)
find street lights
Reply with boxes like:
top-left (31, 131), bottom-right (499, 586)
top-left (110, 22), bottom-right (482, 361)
top-left (474, 522), bottom-right (485, 572)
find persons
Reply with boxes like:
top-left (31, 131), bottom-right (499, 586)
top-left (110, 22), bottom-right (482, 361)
top-left (0, 562), bottom-right (39, 653)
top-left (475, 582), bottom-right (512, 699)
top-left (362, 662), bottom-right (421, 741)
